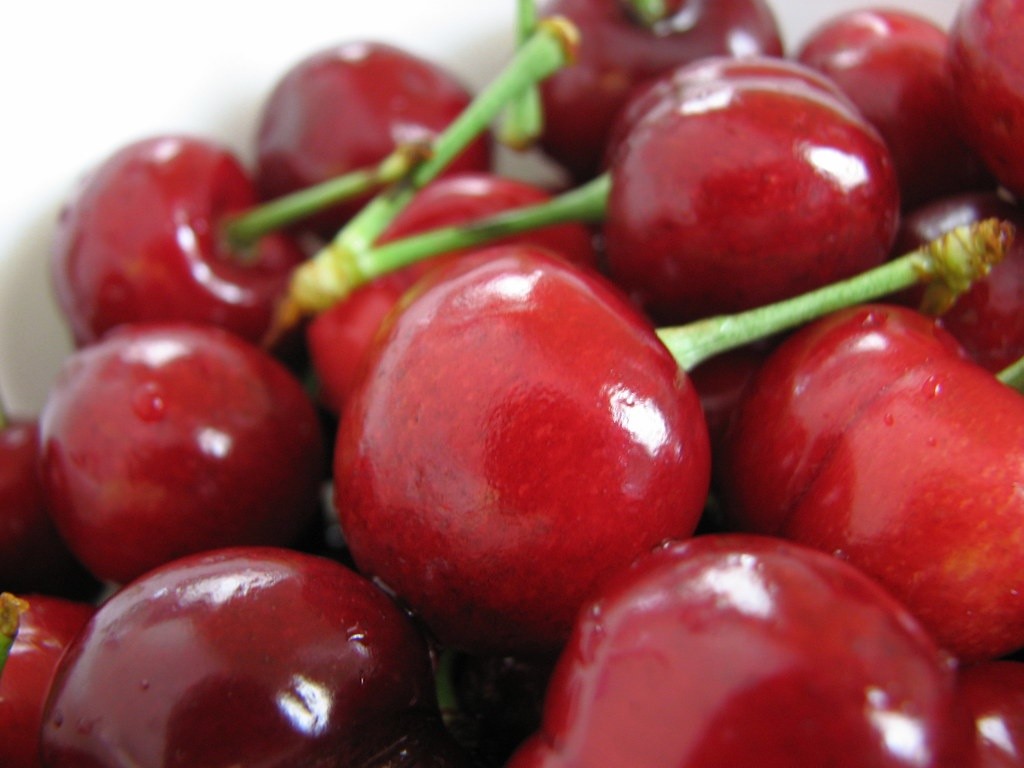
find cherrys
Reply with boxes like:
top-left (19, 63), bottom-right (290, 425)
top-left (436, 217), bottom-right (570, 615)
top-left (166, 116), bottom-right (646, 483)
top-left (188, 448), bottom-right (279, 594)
top-left (1, 4), bottom-right (1024, 767)
